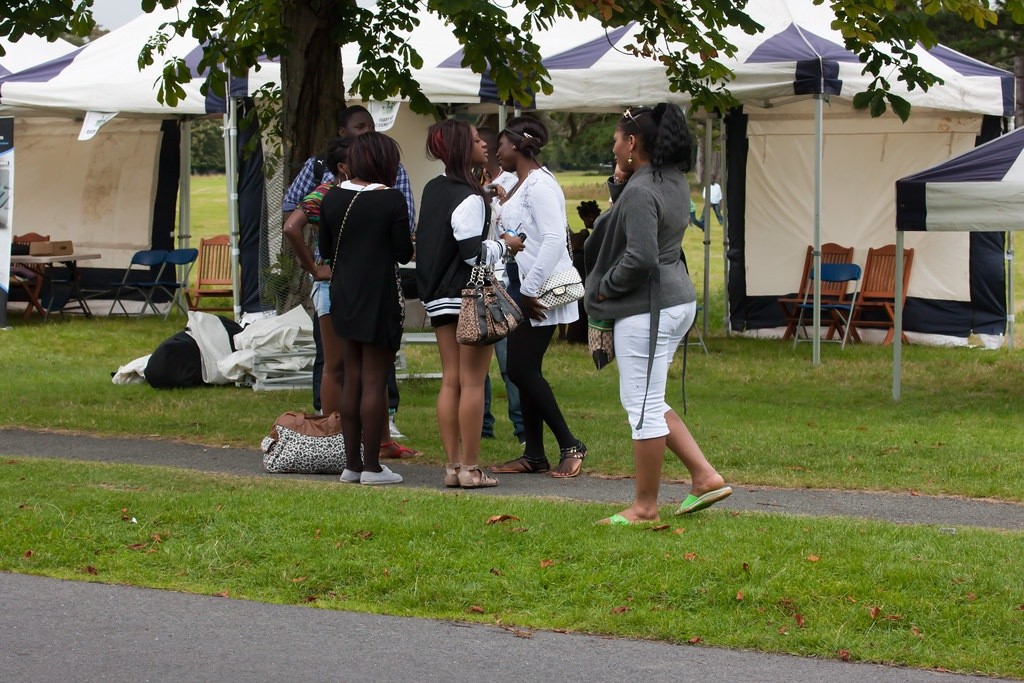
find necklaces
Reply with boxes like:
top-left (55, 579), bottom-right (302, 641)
top-left (510, 182), bottom-right (519, 198)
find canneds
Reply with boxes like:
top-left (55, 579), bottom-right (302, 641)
top-left (506, 229), bottom-right (517, 236)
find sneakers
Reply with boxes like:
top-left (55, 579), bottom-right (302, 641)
top-left (360, 464), bottom-right (403, 485)
top-left (340, 469), bottom-right (361, 483)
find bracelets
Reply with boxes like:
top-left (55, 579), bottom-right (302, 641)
top-left (613, 175), bottom-right (630, 183)
top-left (501, 237), bottom-right (512, 264)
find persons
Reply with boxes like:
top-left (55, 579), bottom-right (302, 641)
top-left (490, 118), bottom-right (590, 480)
top-left (280, 104), bottom-right (414, 440)
top-left (281, 138), bottom-right (424, 463)
top-left (414, 119), bottom-right (526, 489)
top-left (467, 113), bottom-right (530, 445)
top-left (698, 174), bottom-right (725, 226)
top-left (316, 132), bottom-right (415, 485)
top-left (583, 102), bottom-right (732, 531)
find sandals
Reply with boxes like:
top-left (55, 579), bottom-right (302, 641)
top-left (492, 455), bottom-right (550, 472)
top-left (458, 464), bottom-right (499, 489)
top-left (378, 441), bottom-right (423, 461)
top-left (444, 462), bottom-right (462, 488)
top-left (553, 440), bottom-right (588, 478)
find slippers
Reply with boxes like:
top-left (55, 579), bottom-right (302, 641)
top-left (674, 486), bottom-right (732, 516)
top-left (597, 513), bottom-right (661, 526)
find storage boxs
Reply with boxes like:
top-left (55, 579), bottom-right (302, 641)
top-left (29, 240), bottom-right (74, 256)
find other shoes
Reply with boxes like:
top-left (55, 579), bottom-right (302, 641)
top-left (517, 432), bottom-right (526, 446)
top-left (388, 408), bottom-right (405, 439)
top-left (481, 432), bottom-right (496, 440)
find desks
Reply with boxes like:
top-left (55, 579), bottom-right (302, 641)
top-left (10, 253), bottom-right (102, 324)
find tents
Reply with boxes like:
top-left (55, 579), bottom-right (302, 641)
top-left (0, 0), bottom-right (1023, 402)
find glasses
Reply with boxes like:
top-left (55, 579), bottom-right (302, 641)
top-left (622, 105), bottom-right (641, 129)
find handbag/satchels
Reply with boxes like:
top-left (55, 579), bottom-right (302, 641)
top-left (536, 266), bottom-right (585, 309)
top-left (261, 410), bottom-right (364, 473)
top-left (456, 243), bottom-right (524, 346)
top-left (587, 315), bottom-right (615, 371)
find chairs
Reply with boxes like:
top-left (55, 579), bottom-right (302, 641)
top-left (792, 263), bottom-right (862, 351)
top-left (13, 233), bottom-right (50, 320)
top-left (138, 248), bottom-right (199, 322)
top-left (184, 234), bottom-right (241, 311)
top-left (831, 244), bottom-right (915, 347)
top-left (106, 249), bottom-right (168, 319)
top-left (778, 243), bottom-right (854, 341)
